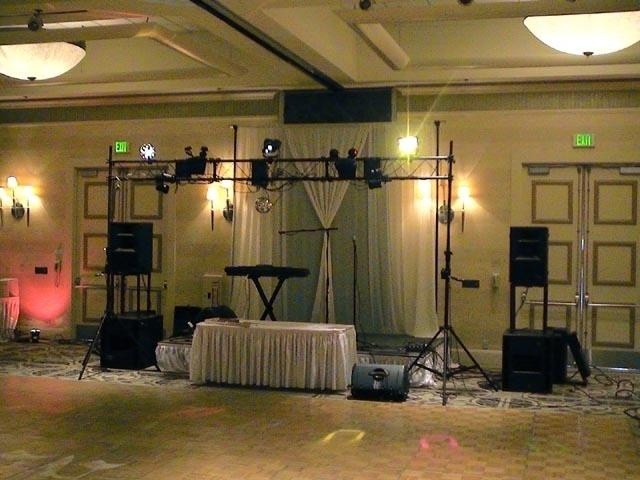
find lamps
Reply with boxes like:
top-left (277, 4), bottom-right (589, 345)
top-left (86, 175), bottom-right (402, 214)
top-left (521, 10), bottom-right (640, 58)
top-left (0, 40), bottom-right (88, 83)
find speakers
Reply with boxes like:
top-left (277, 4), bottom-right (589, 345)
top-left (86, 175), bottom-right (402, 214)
top-left (173, 305), bottom-right (201, 337)
top-left (350, 363), bottom-right (408, 401)
top-left (501, 329), bottom-right (553, 397)
top-left (100, 310), bottom-right (164, 371)
top-left (195, 307), bottom-right (238, 331)
top-left (509, 227), bottom-right (550, 286)
top-left (105, 221), bottom-right (154, 276)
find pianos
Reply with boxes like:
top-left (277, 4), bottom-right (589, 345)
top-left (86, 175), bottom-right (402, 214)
top-left (223, 264), bottom-right (311, 321)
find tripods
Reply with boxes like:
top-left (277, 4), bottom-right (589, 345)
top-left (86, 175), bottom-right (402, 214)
top-left (408, 254), bottom-right (499, 407)
top-left (79, 270), bottom-right (161, 379)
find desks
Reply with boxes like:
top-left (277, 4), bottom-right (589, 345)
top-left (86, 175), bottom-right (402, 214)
top-left (194, 316), bottom-right (357, 392)
